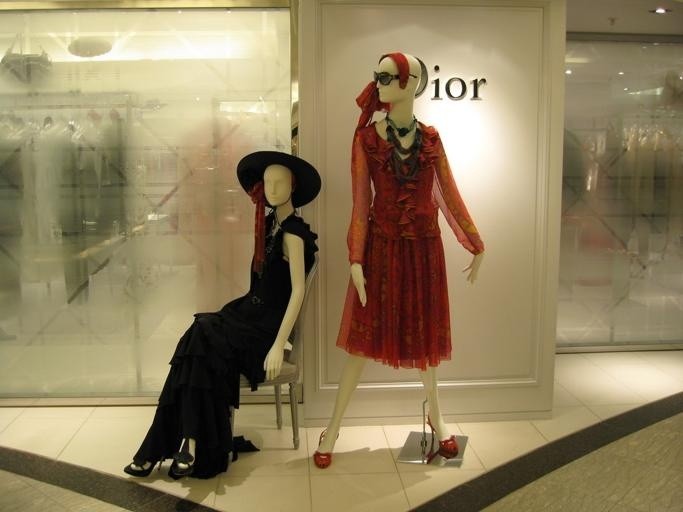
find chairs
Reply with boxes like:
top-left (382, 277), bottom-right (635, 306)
top-left (229, 252), bottom-right (321, 451)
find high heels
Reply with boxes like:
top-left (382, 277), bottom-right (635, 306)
top-left (124, 444), bottom-right (166, 477)
top-left (313, 428), bottom-right (339, 469)
top-left (428, 413), bottom-right (458, 459)
top-left (168, 434), bottom-right (198, 481)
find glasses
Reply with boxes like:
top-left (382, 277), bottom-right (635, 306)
top-left (373, 71), bottom-right (418, 85)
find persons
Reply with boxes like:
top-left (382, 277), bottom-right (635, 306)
top-left (312, 53), bottom-right (484, 468)
top-left (122, 150), bottom-right (320, 480)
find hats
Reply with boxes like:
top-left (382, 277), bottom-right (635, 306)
top-left (236, 151), bottom-right (322, 209)
